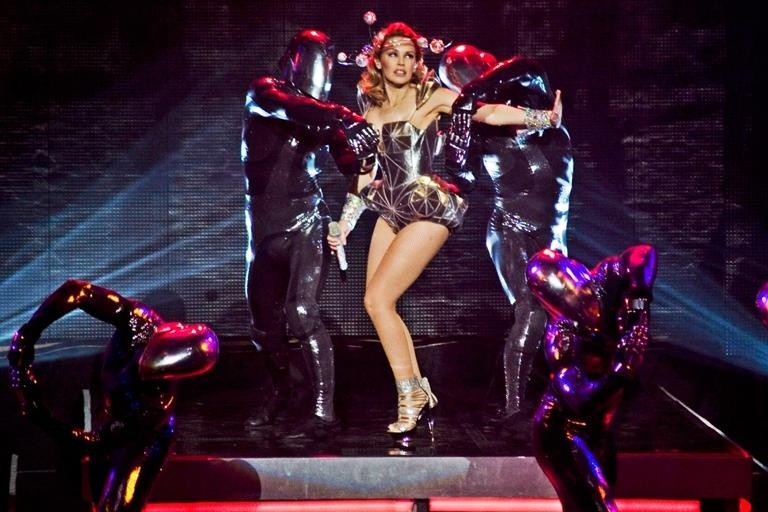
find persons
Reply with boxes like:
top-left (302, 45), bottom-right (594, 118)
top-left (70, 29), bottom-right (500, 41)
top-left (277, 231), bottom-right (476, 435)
top-left (239, 29), bottom-right (381, 450)
top-left (0, 279), bottom-right (220, 512)
top-left (327, 22), bottom-right (563, 434)
top-left (532, 244), bottom-right (658, 508)
top-left (438, 44), bottom-right (573, 425)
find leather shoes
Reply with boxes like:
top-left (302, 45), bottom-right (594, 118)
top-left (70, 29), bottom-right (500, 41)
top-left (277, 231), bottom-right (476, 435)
top-left (475, 399), bottom-right (533, 437)
top-left (245, 388), bottom-right (340, 442)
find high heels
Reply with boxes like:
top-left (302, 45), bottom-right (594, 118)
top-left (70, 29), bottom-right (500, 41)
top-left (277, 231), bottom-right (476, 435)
top-left (387, 377), bottom-right (438, 435)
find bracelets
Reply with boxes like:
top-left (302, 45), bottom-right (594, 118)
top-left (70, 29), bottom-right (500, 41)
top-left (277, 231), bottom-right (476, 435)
top-left (525, 108), bottom-right (552, 132)
top-left (339, 200), bottom-right (364, 228)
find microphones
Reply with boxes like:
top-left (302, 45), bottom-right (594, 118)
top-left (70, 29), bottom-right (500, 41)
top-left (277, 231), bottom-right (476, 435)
top-left (328, 222), bottom-right (348, 271)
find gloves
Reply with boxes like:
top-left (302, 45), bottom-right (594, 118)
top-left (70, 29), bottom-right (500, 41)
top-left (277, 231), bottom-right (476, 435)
top-left (344, 118), bottom-right (380, 157)
top-left (7, 354), bottom-right (44, 419)
top-left (446, 90), bottom-right (478, 178)
top-left (618, 299), bottom-right (651, 365)
